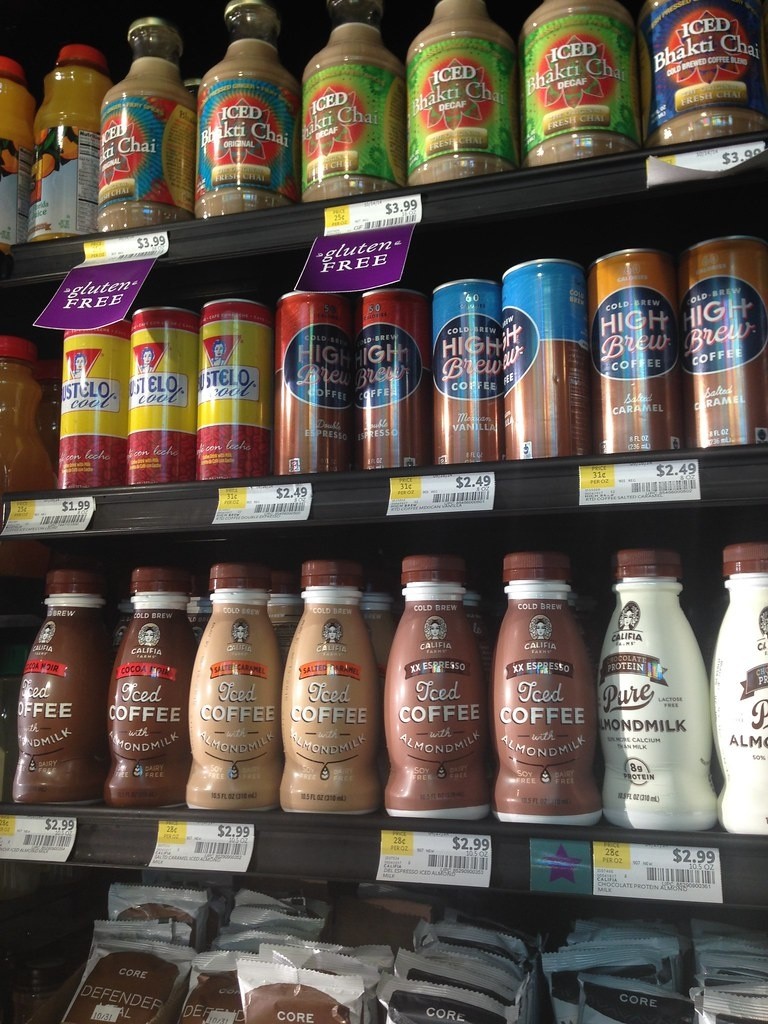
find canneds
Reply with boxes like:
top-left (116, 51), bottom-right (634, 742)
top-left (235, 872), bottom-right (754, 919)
top-left (56, 233), bottom-right (768, 491)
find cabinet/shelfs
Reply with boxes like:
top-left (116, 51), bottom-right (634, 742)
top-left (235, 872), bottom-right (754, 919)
top-left (0, 126), bottom-right (767, 919)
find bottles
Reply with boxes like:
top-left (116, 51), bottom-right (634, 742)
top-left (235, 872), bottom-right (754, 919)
top-left (193, 0), bottom-right (301, 220)
top-left (299, 0), bottom-right (407, 203)
top-left (1, 331), bottom-right (64, 497)
top-left (516, 0), bottom-right (643, 169)
top-left (379, 539), bottom-right (768, 836)
top-left (635, 0), bottom-right (768, 150)
top-left (0, 0), bottom-right (206, 252)
top-left (0, 949), bottom-right (66, 1024)
top-left (0, 536), bottom-right (401, 816)
top-left (404, 0), bottom-right (521, 188)
